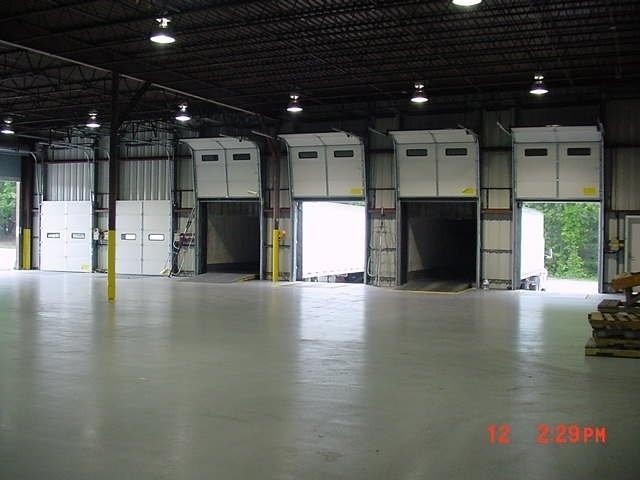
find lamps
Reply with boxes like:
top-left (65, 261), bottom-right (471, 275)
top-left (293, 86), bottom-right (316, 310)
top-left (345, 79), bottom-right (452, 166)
top-left (410, 81), bottom-right (429, 103)
top-left (175, 100), bottom-right (192, 122)
top-left (85, 108), bottom-right (101, 127)
top-left (286, 93), bottom-right (303, 113)
top-left (0, 115), bottom-right (15, 135)
top-left (529, 73), bottom-right (549, 95)
top-left (150, 7), bottom-right (177, 46)
